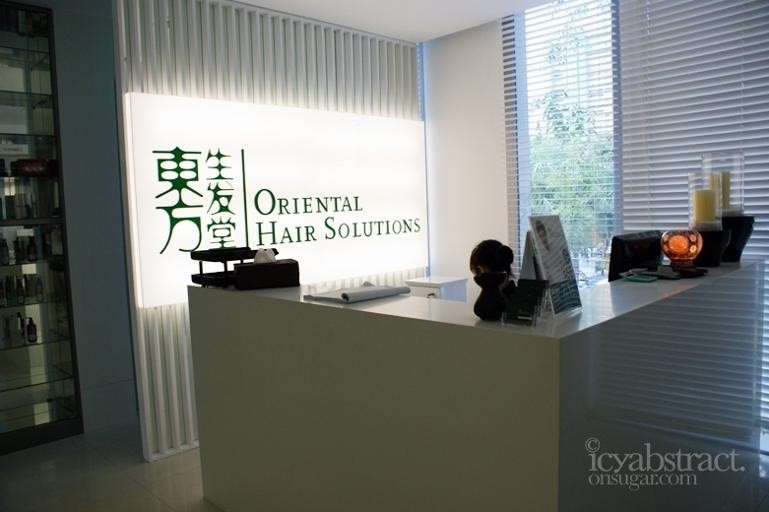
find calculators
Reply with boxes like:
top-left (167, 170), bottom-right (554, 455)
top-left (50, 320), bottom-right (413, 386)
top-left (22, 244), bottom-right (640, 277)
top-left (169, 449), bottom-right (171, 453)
top-left (619, 272), bottom-right (658, 282)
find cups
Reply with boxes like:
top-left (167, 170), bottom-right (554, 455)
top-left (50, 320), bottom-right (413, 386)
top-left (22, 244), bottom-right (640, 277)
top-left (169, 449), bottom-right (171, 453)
top-left (690, 151), bottom-right (756, 268)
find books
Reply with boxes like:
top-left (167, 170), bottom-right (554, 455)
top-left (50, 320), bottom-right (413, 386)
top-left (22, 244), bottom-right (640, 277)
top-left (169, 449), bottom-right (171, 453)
top-left (300, 281), bottom-right (409, 304)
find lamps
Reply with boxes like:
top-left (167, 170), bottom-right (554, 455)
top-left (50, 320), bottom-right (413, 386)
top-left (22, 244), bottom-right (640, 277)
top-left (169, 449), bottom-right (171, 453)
top-left (655, 228), bottom-right (710, 280)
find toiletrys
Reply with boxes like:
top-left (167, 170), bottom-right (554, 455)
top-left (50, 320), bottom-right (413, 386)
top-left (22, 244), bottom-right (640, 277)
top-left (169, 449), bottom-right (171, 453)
top-left (0, 190), bottom-right (64, 344)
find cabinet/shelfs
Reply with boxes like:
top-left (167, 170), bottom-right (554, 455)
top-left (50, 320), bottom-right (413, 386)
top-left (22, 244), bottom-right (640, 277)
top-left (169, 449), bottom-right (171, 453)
top-left (404, 274), bottom-right (469, 302)
top-left (0, 0), bottom-right (87, 460)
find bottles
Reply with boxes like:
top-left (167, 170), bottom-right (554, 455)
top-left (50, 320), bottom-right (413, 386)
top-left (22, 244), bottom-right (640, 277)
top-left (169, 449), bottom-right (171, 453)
top-left (0, 232), bottom-right (45, 343)
top-left (472, 271), bottom-right (507, 321)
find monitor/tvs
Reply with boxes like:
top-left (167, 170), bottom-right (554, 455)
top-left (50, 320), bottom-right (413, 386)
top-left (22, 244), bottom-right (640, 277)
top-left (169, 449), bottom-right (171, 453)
top-left (608, 230), bottom-right (665, 282)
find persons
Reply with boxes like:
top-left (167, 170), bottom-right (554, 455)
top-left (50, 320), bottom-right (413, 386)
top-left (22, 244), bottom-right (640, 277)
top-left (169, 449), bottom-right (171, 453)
top-left (467, 239), bottom-right (519, 298)
top-left (534, 219), bottom-right (568, 287)
